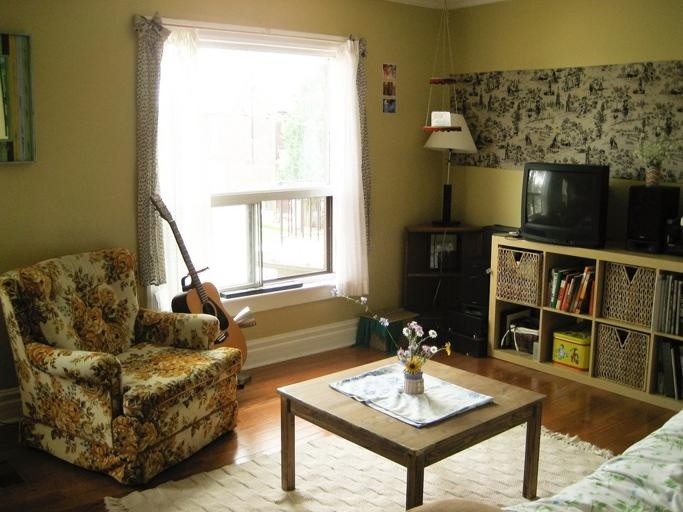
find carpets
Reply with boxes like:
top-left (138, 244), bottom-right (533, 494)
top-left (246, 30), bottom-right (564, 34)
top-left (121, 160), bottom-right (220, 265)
top-left (100, 418), bottom-right (618, 512)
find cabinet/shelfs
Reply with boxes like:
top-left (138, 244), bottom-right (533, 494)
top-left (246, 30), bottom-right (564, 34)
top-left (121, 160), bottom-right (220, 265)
top-left (483, 229), bottom-right (683, 414)
top-left (399, 223), bottom-right (483, 322)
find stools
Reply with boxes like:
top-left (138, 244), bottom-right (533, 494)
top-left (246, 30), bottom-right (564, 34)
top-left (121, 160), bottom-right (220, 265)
top-left (350, 303), bottom-right (422, 357)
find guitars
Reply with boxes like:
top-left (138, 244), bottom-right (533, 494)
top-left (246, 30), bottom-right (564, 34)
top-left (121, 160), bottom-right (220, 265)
top-left (149, 190), bottom-right (248, 372)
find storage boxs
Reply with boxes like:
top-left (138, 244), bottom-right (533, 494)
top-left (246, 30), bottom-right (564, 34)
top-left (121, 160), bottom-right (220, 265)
top-left (551, 327), bottom-right (592, 373)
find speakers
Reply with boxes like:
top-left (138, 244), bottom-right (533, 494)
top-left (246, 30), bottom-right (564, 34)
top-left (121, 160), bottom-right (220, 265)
top-left (405, 224), bottom-right (468, 315)
top-left (628, 184), bottom-right (680, 254)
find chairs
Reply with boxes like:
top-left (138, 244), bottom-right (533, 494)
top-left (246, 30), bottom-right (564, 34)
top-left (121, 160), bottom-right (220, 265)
top-left (0, 246), bottom-right (244, 492)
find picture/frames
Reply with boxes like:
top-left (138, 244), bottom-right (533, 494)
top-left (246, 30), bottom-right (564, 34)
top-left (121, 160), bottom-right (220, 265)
top-left (379, 60), bottom-right (399, 118)
top-left (0, 31), bottom-right (38, 166)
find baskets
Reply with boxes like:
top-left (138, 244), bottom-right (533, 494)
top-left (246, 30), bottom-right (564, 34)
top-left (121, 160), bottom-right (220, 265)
top-left (601, 260), bottom-right (656, 328)
top-left (495, 247), bottom-right (542, 307)
top-left (593, 323), bottom-right (650, 392)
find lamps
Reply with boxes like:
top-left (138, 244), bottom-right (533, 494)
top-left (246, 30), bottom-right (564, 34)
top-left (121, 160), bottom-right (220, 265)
top-left (421, 108), bottom-right (481, 228)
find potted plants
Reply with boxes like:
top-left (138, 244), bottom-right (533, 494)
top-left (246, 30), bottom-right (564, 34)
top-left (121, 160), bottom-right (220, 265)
top-left (644, 155), bottom-right (664, 188)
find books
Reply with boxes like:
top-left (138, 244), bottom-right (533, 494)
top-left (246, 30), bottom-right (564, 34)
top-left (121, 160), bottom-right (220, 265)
top-left (657, 274), bottom-right (683, 401)
top-left (546, 265), bottom-right (595, 315)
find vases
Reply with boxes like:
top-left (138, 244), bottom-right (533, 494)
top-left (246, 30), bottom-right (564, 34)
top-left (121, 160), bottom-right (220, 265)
top-left (400, 368), bottom-right (426, 396)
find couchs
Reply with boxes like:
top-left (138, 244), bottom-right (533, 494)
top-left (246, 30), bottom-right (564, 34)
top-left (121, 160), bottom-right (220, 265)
top-left (397, 406), bottom-right (683, 511)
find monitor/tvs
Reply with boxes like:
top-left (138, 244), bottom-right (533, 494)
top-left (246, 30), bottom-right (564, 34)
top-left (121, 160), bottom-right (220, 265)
top-left (520, 161), bottom-right (609, 248)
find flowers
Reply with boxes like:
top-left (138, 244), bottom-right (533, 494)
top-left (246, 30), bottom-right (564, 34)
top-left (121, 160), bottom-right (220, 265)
top-left (328, 284), bottom-right (453, 375)
